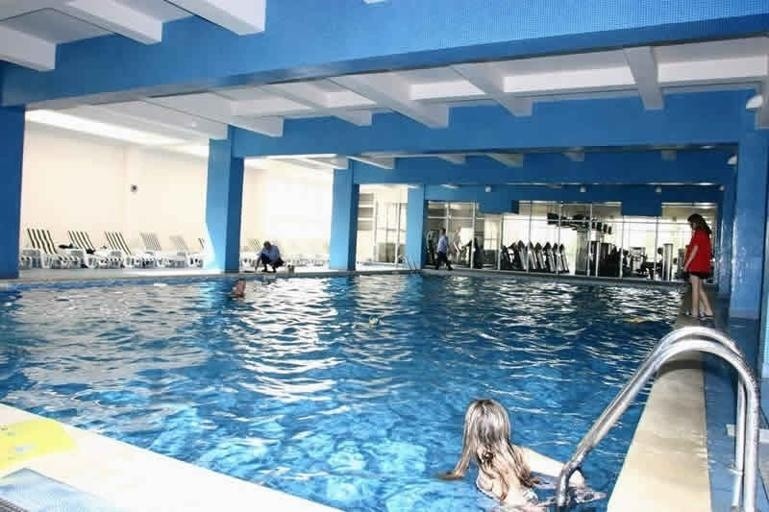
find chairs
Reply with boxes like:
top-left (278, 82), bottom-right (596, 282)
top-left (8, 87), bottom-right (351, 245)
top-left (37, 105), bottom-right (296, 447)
top-left (19, 226), bottom-right (371, 270)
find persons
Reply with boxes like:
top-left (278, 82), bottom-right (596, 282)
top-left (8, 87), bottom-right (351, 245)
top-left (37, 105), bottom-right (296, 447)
top-left (434, 396), bottom-right (608, 512)
top-left (682, 213), bottom-right (717, 319)
top-left (435, 228), bottom-right (455, 272)
top-left (231, 276), bottom-right (251, 299)
top-left (254, 241), bottom-right (284, 273)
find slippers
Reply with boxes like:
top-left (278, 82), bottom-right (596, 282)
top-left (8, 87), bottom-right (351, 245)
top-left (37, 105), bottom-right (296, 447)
top-left (686, 311), bottom-right (699, 316)
top-left (700, 312), bottom-right (714, 319)
